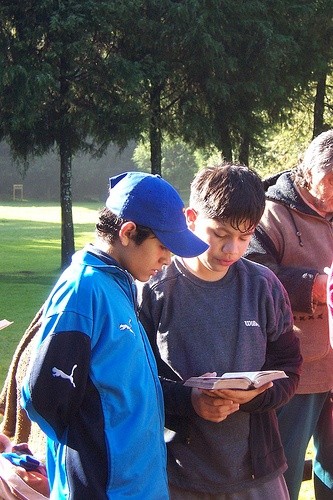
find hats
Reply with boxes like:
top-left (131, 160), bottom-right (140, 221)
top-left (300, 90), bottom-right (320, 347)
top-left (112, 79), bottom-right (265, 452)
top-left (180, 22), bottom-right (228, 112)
top-left (106, 171), bottom-right (210, 259)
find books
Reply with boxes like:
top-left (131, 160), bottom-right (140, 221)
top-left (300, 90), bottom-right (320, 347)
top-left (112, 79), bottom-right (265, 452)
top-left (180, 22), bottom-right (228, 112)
top-left (183, 371), bottom-right (289, 391)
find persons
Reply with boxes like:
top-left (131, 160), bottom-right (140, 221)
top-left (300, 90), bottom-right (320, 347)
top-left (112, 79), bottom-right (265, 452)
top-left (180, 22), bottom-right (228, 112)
top-left (241, 129), bottom-right (332, 500)
top-left (135, 164), bottom-right (302, 500)
top-left (21, 171), bottom-right (209, 500)
